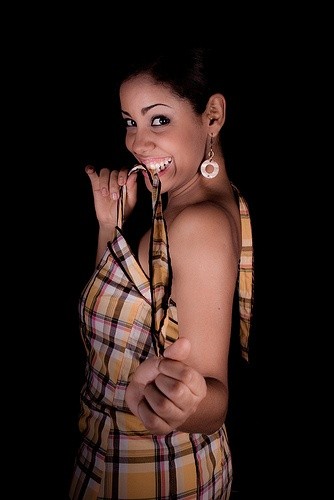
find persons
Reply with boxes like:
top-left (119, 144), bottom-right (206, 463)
top-left (68, 33), bottom-right (253, 499)
